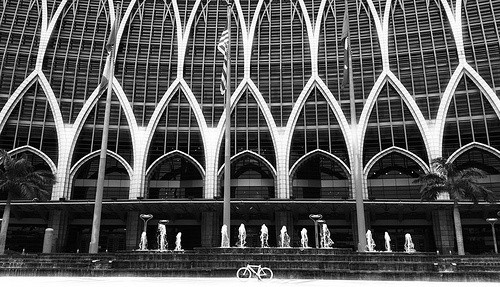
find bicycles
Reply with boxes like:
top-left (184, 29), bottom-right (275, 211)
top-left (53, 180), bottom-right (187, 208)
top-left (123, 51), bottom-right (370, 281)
top-left (236, 260), bottom-right (273, 281)
